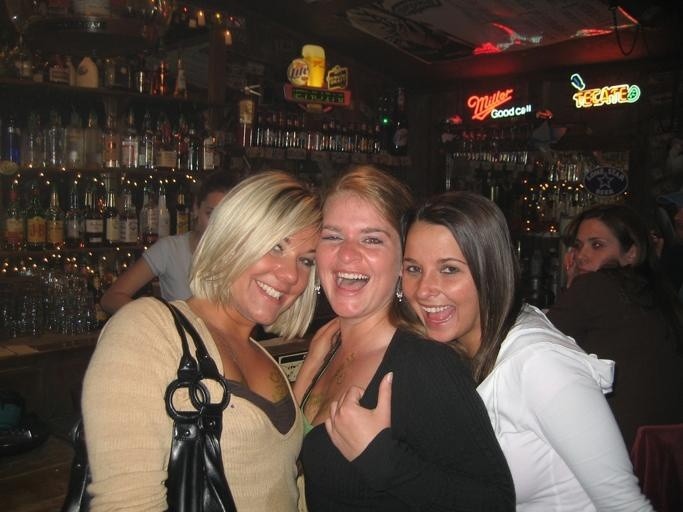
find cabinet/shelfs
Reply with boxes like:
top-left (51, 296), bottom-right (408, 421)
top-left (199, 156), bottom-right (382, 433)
top-left (0, 1), bottom-right (220, 510)
top-left (220, 31), bottom-right (408, 212)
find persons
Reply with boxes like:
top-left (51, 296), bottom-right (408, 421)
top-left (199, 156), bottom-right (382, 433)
top-left (78, 169), bottom-right (323, 512)
top-left (399, 190), bottom-right (656, 512)
top-left (545, 202), bottom-right (682, 460)
top-left (298, 163), bottom-right (517, 511)
top-left (99, 173), bottom-right (239, 314)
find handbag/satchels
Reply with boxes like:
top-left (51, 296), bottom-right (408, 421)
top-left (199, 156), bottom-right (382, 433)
top-left (60, 403), bottom-right (237, 511)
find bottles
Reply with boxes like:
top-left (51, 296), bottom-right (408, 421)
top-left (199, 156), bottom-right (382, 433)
top-left (94, 271), bottom-right (111, 329)
top-left (451, 126), bottom-right (528, 164)
top-left (253, 107), bottom-right (383, 154)
top-left (1, 171), bottom-right (222, 251)
top-left (1, 30), bottom-right (187, 101)
top-left (488, 150), bottom-right (587, 310)
top-left (0, 88), bottom-right (221, 171)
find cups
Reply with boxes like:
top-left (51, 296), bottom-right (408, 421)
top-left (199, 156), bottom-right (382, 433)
top-left (0, 272), bottom-right (92, 336)
